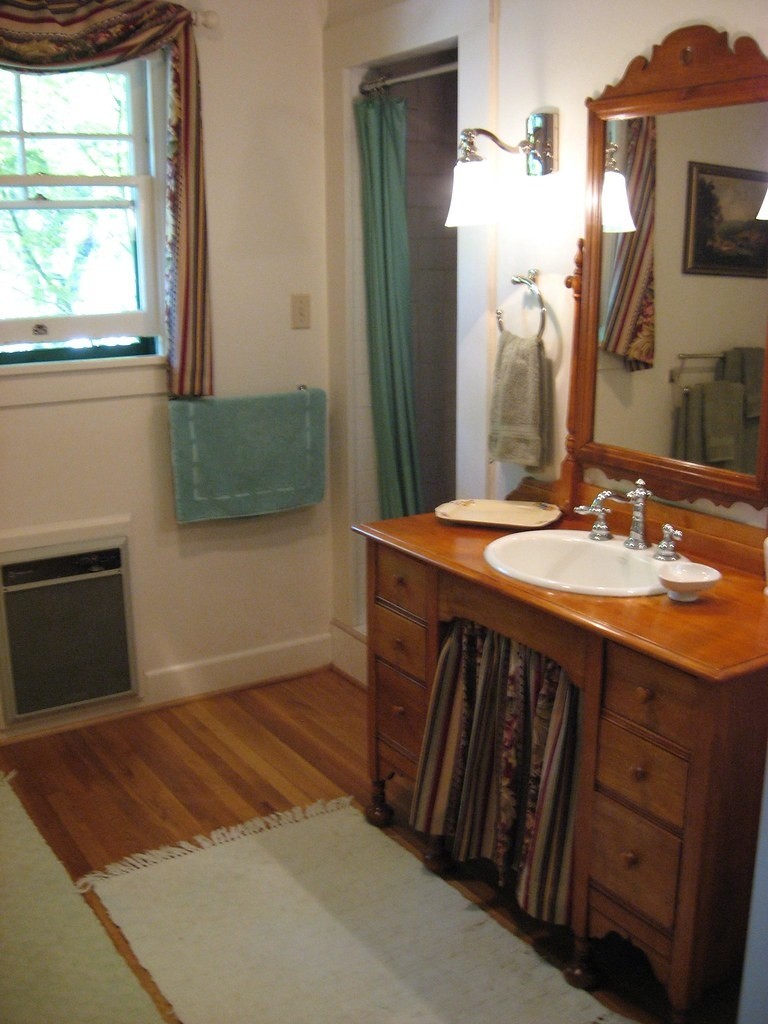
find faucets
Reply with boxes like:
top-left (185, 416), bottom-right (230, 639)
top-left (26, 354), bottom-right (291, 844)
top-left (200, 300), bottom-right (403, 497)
top-left (622, 479), bottom-right (654, 550)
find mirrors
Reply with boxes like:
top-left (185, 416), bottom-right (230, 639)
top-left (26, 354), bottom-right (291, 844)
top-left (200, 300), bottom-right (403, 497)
top-left (567, 25), bottom-right (768, 511)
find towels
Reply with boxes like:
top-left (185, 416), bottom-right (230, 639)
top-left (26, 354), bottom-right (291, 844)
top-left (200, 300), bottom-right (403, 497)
top-left (484, 329), bottom-right (549, 475)
top-left (672, 347), bottom-right (764, 476)
top-left (169, 387), bottom-right (325, 526)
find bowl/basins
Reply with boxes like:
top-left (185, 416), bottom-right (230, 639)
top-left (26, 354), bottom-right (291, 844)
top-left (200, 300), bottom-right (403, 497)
top-left (656, 562), bottom-right (723, 602)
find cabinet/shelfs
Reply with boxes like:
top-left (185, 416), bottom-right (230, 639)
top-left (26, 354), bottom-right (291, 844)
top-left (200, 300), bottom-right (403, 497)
top-left (348, 476), bottom-right (768, 1019)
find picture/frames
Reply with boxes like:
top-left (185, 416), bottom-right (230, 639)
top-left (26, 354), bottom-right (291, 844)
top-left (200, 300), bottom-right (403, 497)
top-left (682, 161), bottom-right (768, 278)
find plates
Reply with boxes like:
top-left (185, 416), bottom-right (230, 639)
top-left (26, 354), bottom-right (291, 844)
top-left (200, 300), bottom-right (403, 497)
top-left (437, 499), bottom-right (562, 527)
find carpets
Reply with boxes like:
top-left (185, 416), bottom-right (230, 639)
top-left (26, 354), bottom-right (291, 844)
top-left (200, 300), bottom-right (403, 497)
top-left (0, 763), bottom-right (169, 1024)
top-left (73, 794), bottom-right (638, 1024)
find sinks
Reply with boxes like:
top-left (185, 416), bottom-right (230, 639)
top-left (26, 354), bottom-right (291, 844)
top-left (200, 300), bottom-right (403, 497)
top-left (482, 530), bottom-right (693, 598)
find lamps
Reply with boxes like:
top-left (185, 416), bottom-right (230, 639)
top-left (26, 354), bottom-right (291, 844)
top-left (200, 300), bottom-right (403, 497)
top-left (600, 142), bottom-right (637, 232)
top-left (444, 110), bottom-right (560, 227)
